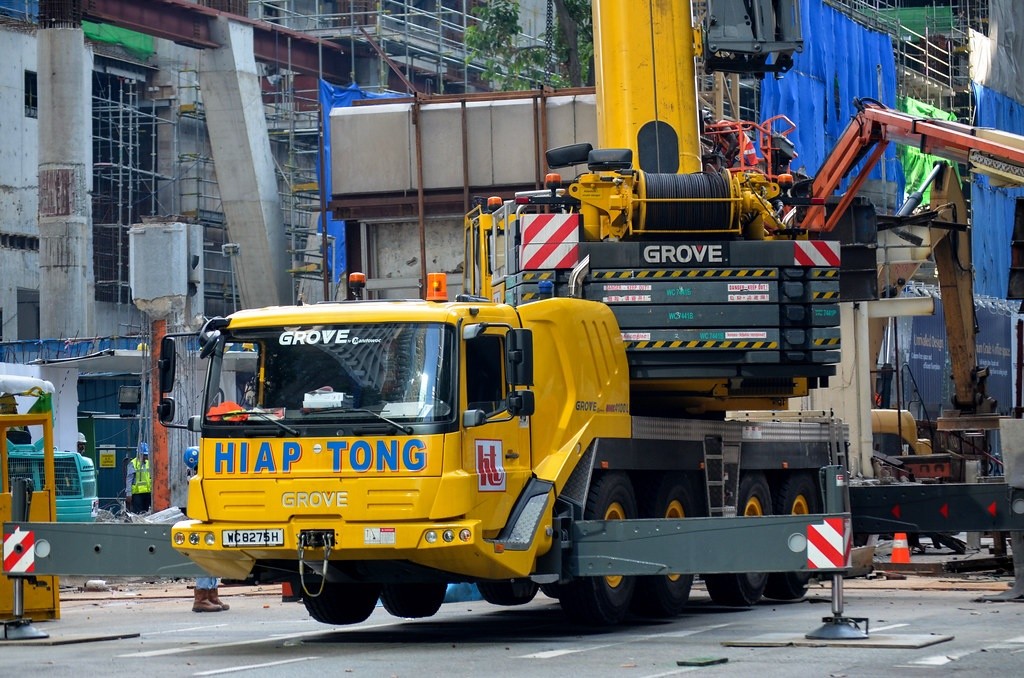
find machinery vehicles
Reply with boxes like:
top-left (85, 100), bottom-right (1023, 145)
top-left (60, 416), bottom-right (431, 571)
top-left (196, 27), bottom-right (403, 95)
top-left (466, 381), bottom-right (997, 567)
top-left (156, 0), bottom-right (1024, 625)
top-left (0, 376), bottom-right (100, 523)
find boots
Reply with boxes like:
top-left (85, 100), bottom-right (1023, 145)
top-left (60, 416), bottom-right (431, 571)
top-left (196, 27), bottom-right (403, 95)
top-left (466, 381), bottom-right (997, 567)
top-left (206, 589), bottom-right (230, 611)
top-left (191, 587), bottom-right (223, 613)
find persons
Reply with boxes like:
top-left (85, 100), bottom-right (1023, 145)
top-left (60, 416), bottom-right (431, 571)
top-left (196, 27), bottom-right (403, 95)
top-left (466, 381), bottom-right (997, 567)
top-left (124, 444), bottom-right (152, 514)
top-left (77, 432), bottom-right (87, 456)
top-left (702, 114), bottom-right (759, 176)
top-left (0, 392), bottom-right (31, 443)
top-left (190, 577), bottom-right (230, 613)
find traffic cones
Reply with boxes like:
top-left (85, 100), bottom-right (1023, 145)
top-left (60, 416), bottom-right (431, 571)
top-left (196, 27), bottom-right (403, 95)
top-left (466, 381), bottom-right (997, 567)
top-left (886, 532), bottom-right (911, 572)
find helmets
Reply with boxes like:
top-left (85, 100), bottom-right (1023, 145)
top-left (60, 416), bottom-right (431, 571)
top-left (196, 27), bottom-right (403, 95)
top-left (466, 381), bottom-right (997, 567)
top-left (703, 109), bottom-right (712, 119)
top-left (183, 445), bottom-right (200, 471)
top-left (136, 442), bottom-right (149, 456)
